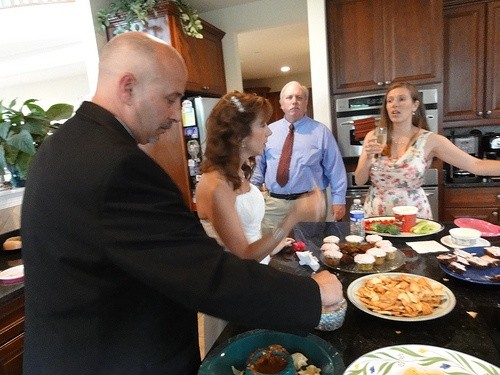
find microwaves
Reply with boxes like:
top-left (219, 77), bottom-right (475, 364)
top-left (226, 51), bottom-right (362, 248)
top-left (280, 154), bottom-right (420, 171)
top-left (335, 88), bottom-right (439, 158)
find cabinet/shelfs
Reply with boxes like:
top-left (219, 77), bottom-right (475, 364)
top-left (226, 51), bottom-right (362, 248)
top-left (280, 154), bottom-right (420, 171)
top-left (104, 0), bottom-right (227, 221)
top-left (325, 0), bottom-right (500, 129)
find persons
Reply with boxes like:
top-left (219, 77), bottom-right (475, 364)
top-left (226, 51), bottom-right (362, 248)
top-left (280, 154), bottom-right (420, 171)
top-left (20, 32), bottom-right (343, 375)
top-left (354, 82), bottom-right (500, 220)
top-left (249, 81), bottom-right (346, 239)
top-left (194, 89), bottom-right (324, 354)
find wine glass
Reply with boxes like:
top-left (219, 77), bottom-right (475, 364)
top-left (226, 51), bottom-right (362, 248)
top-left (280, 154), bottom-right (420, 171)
top-left (371, 126), bottom-right (387, 171)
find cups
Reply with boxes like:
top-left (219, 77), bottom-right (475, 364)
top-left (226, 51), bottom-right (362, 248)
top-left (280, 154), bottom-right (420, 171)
top-left (392, 205), bottom-right (418, 233)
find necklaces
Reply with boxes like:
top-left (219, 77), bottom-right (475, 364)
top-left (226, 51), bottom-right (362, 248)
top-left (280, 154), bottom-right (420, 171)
top-left (393, 137), bottom-right (409, 144)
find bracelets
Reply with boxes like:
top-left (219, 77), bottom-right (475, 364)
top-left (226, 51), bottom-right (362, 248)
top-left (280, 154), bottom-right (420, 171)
top-left (273, 225), bottom-right (285, 241)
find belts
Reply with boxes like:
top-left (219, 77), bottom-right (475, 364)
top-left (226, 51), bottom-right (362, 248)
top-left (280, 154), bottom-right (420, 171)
top-left (269, 191), bottom-right (309, 200)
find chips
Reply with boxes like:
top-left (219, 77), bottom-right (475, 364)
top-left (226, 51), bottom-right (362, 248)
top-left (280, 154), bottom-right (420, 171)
top-left (354, 275), bottom-right (447, 317)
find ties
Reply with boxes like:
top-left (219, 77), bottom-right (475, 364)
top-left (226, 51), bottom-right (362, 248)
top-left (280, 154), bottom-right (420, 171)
top-left (276, 122), bottom-right (295, 188)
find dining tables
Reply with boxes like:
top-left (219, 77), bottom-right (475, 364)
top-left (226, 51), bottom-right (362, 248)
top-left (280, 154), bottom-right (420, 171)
top-left (203, 222), bottom-right (500, 375)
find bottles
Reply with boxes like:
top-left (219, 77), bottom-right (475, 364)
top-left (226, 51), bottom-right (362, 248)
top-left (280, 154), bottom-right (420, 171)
top-left (349, 199), bottom-right (365, 238)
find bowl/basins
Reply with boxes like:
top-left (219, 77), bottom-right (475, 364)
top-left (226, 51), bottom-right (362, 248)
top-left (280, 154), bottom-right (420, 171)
top-left (449, 228), bottom-right (481, 246)
top-left (197, 328), bottom-right (345, 375)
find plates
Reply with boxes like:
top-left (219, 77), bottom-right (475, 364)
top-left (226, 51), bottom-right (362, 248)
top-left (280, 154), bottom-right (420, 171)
top-left (347, 273), bottom-right (456, 322)
top-left (343, 344), bottom-right (500, 375)
top-left (440, 235), bottom-right (491, 248)
top-left (454, 217), bottom-right (500, 237)
top-left (354, 216), bottom-right (445, 237)
top-left (319, 248), bottom-right (406, 273)
top-left (438, 247), bottom-right (500, 285)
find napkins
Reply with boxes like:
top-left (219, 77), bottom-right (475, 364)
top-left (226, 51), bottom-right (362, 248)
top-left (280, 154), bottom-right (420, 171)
top-left (404, 240), bottom-right (449, 254)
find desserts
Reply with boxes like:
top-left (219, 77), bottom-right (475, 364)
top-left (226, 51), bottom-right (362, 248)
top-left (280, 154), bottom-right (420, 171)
top-left (320, 234), bottom-right (397, 270)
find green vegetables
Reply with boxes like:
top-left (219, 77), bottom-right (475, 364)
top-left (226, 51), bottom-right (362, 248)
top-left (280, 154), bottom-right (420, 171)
top-left (410, 221), bottom-right (437, 234)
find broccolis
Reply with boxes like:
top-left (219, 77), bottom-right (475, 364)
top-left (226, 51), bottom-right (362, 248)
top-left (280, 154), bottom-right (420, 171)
top-left (371, 221), bottom-right (400, 235)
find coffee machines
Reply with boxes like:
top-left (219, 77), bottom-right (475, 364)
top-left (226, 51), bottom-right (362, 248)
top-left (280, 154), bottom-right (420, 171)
top-left (446, 134), bottom-right (479, 183)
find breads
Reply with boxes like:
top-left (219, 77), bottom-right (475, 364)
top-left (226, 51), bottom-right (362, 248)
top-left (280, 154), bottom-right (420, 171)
top-left (436, 246), bottom-right (500, 283)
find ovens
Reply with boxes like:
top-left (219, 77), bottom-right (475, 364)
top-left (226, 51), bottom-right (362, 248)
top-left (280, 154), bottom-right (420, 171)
top-left (346, 168), bottom-right (439, 222)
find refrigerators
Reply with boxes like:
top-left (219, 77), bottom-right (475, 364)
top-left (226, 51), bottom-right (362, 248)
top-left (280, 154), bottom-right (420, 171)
top-left (181, 95), bottom-right (221, 201)
